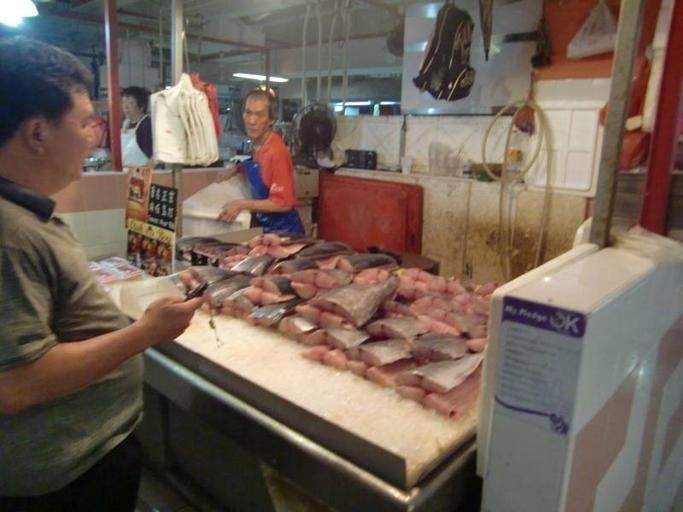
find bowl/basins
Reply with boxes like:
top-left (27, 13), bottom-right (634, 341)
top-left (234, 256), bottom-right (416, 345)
top-left (473, 163), bottom-right (503, 181)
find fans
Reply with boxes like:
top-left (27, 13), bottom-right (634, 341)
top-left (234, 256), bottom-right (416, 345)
top-left (289, 103), bottom-right (337, 169)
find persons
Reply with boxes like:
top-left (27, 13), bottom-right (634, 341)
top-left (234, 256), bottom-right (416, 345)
top-left (215, 89), bottom-right (305, 237)
top-left (0, 34), bottom-right (211, 512)
top-left (118, 85), bottom-right (151, 168)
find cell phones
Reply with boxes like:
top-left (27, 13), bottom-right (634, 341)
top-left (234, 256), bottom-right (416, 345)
top-left (185, 280), bottom-right (208, 300)
